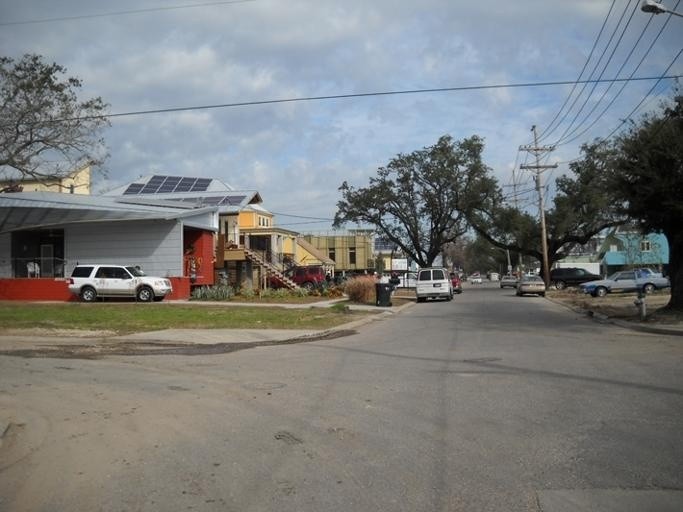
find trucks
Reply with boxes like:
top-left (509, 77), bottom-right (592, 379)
top-left (415, 266), bottom-right (454, 301)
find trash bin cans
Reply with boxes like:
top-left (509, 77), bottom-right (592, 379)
top-left (374, 283), bottom-right (393, 306)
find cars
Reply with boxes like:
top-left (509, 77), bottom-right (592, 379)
top-left (632, 268), bottom-right (663, 279)
top-left (516, 275), bottom-right (546, 298)
top-left (579, 271), bottom-right (670, 297)
top-left (460, 273), bottom-right (500, 284)
top-left (500, 276), bottom-right (517, 288)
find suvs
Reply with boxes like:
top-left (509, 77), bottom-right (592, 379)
top-left (550, 268), bottom-right (604, 290)
top-left (262, 265), bottom-right (325, 297)
top-left (67, 264), bottom-right (172, 301)
top-left (449, 272), bottom-right (463, 295)
